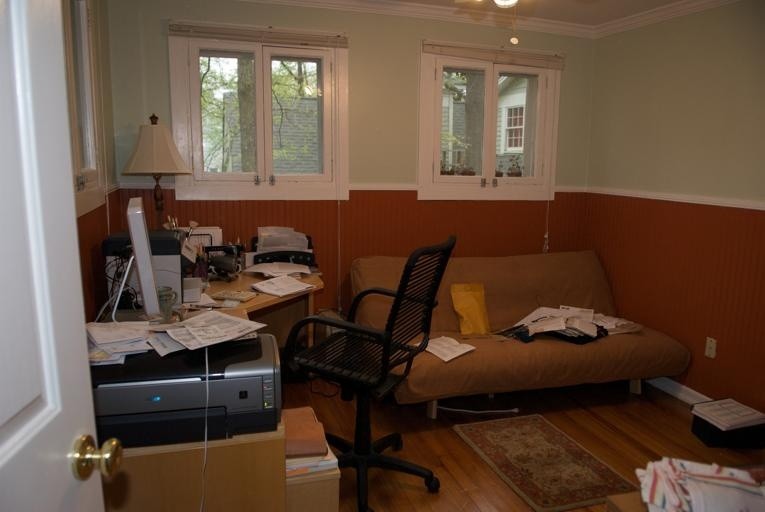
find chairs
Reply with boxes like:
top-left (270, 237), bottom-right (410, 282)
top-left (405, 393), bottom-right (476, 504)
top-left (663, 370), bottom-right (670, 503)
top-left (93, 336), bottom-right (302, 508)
top-left (284, 232), bottom-right (460, 511)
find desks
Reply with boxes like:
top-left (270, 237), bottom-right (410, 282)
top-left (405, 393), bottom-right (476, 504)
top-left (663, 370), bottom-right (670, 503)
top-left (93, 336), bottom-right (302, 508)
top-left (103, 253), bottom-right (325, 347)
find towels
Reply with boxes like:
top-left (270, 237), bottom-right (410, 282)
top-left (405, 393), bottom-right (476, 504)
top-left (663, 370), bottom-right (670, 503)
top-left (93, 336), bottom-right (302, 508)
top-left (633, 454), bottom-right (764, 511)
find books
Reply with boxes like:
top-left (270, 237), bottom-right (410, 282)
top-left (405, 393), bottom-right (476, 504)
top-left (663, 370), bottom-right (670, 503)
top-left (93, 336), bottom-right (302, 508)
top-left (281, 406), bottom-right (339, 477)
top-left (210, 289), bottom-right (256, 302)
top-left (240, 226), bottom-right (314, 297)
top-left (90, 307), bottom-right (269, 366)
top-left (496, 304), bottom-right (644, 345)
top-left (415, 335), bottom-right (477, 362)
top-left (174, 225), bottom-right (223, 257)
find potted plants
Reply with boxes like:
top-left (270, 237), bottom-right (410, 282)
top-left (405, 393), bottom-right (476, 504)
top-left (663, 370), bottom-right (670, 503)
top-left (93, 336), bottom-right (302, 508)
top-left (440, 154), bottom-right (525, 177)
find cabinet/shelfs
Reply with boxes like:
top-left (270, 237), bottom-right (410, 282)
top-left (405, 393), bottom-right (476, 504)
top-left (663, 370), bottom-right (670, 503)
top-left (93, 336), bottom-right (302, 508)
top-left (97, 402), bottom-right (343, 511)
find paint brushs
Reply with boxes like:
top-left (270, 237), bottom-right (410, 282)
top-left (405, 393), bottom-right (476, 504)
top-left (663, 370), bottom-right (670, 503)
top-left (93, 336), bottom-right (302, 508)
top-left (168, 216), bottom-right (193, 241)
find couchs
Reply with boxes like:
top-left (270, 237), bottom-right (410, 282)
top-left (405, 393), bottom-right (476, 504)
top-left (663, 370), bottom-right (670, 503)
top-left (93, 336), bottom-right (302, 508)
top-left (343, 249), bottom-right (691, 419)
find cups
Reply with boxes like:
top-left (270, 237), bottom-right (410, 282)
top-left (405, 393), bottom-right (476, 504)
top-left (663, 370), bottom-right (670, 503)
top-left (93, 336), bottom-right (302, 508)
top-left (157, 286), bottom-right (178, 319)
top-left (192, 260), bottom-right (208, 280)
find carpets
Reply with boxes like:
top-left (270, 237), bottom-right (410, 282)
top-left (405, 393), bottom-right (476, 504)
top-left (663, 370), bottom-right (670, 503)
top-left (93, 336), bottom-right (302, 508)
top-left (452, 411), bottom-right (639, 509)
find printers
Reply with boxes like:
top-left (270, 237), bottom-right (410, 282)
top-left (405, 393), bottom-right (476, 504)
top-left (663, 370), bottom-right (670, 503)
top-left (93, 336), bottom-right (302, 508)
top-left (101, 228), bottom-right (198, 310)
top-left (89, 333), bottom-right (282, 449)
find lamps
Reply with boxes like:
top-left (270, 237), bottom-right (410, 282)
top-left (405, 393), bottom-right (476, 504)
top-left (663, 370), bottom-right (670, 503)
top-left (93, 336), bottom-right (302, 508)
top-left (120, 111), bottom-right (194, 228)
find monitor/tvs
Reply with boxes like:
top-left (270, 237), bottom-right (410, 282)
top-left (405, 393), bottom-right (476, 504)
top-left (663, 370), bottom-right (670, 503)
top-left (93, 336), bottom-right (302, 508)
top-left (110, 196), bottom-right (162, 322)
